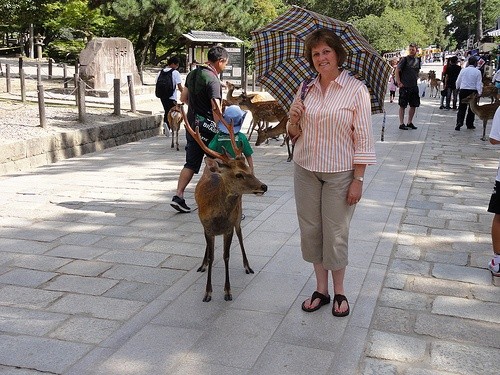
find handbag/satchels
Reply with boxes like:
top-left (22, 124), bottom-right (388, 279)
top-left (193, 111), bottom-right (219, 143)
top-left (441, 89), bottom-right (448, 96)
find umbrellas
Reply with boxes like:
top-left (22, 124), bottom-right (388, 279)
top-left (251, 4), bottom-right (395, 142)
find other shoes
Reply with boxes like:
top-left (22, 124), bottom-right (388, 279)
top-left (446, 106), bottom-right (451, 109)
top-left (399, 123), bottom-right (409, 131)
top-left (162, 121), bottom-right (172, 137)
top-left (406, 122), bottom-right (418, 129)
top-left (453, 105), bottom-right (457, 109)
top-left (439, 106), bottom-right (445, 109)
top-left (467, 125), bottom-right (476, 130)
top-left (454, 122), bottom-right (463, 131)
top-left (390, 100), bottom-right (393, 103)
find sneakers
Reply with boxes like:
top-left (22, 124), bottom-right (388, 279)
top-left (487, 259), bottom-right (500, 276)
top-left (170, 196), bottom-right (191, 213)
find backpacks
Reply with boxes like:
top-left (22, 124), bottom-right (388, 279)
top-left (155, 67), bottom-right (176, 99)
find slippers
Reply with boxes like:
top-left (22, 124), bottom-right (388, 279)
top-left (302, 290), bottom-right (330, 313)
top-left (332, 294), bottom-right (350, 317)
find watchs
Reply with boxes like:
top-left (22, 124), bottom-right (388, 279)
top-left (353, 177), bottom-right (364, 181)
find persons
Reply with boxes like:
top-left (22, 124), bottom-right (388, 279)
top-left (208, 105), bottom-right (254, 173)
top-left (170, 46), bottom-right (229, 212)
top-left (156, 56), bottom-right (184, 137)
top-left (285, 29), bottom-right (378, 318)
top-left (486, 105), bottom-right (500, 277)
top-left (388, 43), bottom-right (483, 130)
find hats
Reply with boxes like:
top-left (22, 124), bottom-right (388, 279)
top-left (217, 104), bottom-right (247, 135)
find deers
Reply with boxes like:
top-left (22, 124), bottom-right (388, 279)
top-left (179, 94), bottom-right (230, 114)
top-left (461, 91), bottom-right (500, 141)
top-left (226, 81), bottom-right (269, 135)
top-left (253, 115), bottom-right (295, 162)
top-left (239, 94), bottom-right (286, 141)
top-left (167, 101), bottom-right (184, 151)
top-left (419, 70), bottom-right (442, 99)
top-left (180, 98), bottom-right (267, 302)
